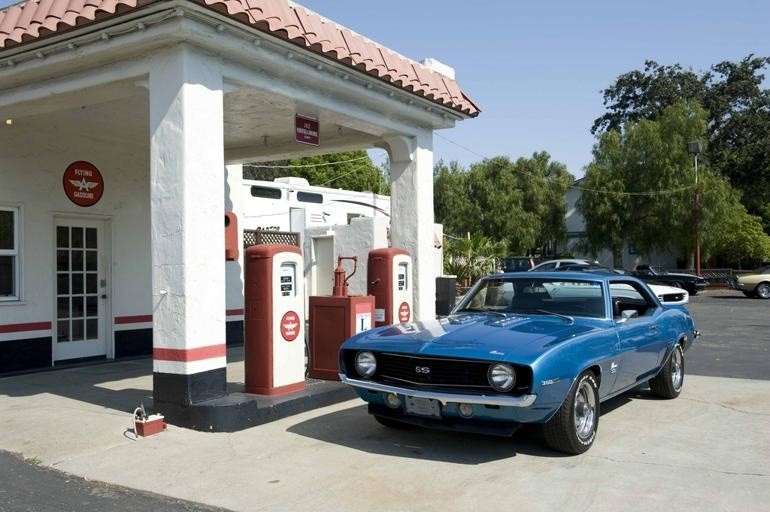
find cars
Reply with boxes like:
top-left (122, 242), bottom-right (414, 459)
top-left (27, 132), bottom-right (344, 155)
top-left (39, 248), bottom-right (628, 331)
top-left (613, 264), bottom-right (711, 297)
top-left (726, 264), bottom-right (770, 299)
top-left (484, 257), bottom-right (692, 310)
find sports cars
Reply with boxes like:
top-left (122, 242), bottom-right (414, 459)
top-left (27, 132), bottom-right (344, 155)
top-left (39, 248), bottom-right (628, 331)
top-left (338, 269), bottom-right (698, 462)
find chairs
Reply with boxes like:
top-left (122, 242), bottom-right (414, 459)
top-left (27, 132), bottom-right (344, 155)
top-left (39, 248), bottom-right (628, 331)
top-left (506, 294), bottom-right (543, 314)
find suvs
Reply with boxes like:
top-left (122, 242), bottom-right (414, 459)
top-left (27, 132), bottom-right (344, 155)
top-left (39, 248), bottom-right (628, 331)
top-left (500, 255), bottom-right (541, 273)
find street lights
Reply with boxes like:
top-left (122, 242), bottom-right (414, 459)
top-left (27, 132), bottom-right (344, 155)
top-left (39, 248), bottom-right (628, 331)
top-left (687, 139), bottom-right (704, 277)
